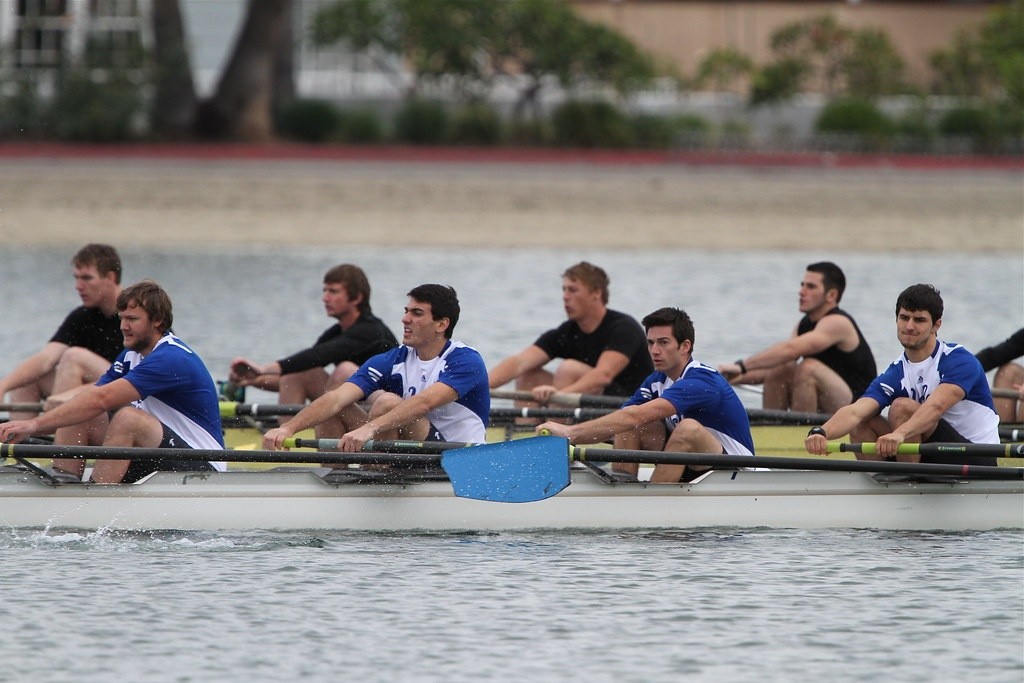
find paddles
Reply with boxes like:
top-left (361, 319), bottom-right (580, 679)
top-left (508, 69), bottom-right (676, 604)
top-left (723, 372), bottom-right (765, 394)
top-left (0, 401), bottom-right (44, 415)
top-left (823, 441), bottom-right (1024, 458)
top-left (991, 386), bottom-right (1024, 402)
top-left (488, 388), bottom-right (831, 427)
top-left (219, 398), bottom-right (616, 422)
top-left (225, 362), bottom-right (248, 401)
top-left (282, 436), bottom-right (1023, 482)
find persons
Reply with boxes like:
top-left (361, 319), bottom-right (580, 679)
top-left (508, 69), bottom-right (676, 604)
top-left (262, 282), bottom-right (491, 467)
top-left (717, 261), bottom-right (878, 414)
top-left (488, 262), bottom-right (654, 425)
top-left (804, 284), bottom-right (1002, 467)
top-left (535, 308), bottom-right (756, 484)
top-left (229, 264), bottom-right (399, 424)
top-left (0, 246), bottom-right (227, 485)
top-left (974, 328), bottom-right (1024, 424)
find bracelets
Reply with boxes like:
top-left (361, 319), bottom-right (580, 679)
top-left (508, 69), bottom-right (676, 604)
top-left (736, 359), bottom-right (746, 373)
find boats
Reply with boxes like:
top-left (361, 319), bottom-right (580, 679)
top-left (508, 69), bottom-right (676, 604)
top-left (8, 401), bottom-right (1024, 472)
top-left (1, 470), bottom-right (1024, 533)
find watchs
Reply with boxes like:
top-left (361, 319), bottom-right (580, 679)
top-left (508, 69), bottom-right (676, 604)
top-left (807, 427), bottom-right (827, 437)
